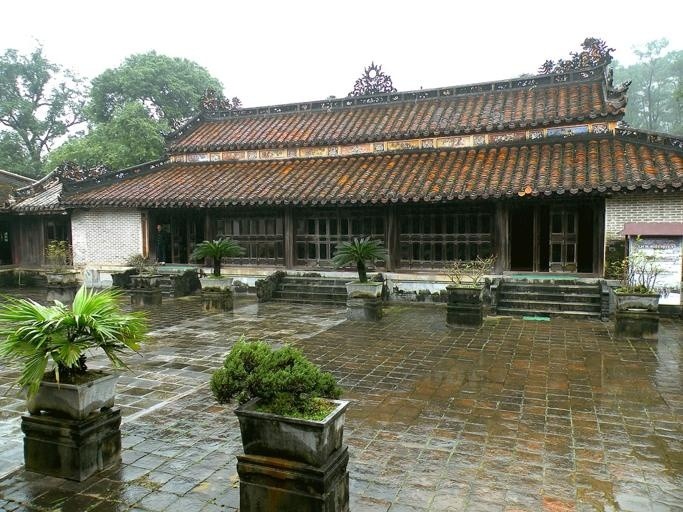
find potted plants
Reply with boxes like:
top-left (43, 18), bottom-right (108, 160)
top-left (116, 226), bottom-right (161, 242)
top-left (210, 334), bottom-right (352, 466)
top-left (329, 236), bottom-right (394, 299)
top-left (125, 255), bottom-right (165, 290)
top-left (443, 252), bottom-right (503, 304)
top-left (38, 240), bottom-right (86, 287)
top-left (185, 237), bottom-right (247, 291)
top-left (0, 280), bottom-right (151, 422)
top-left (610, 230), bottom-right (671, 310)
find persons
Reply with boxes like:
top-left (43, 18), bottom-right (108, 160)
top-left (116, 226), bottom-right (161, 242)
top-left (153, 224), bottom-right (168, 265)
